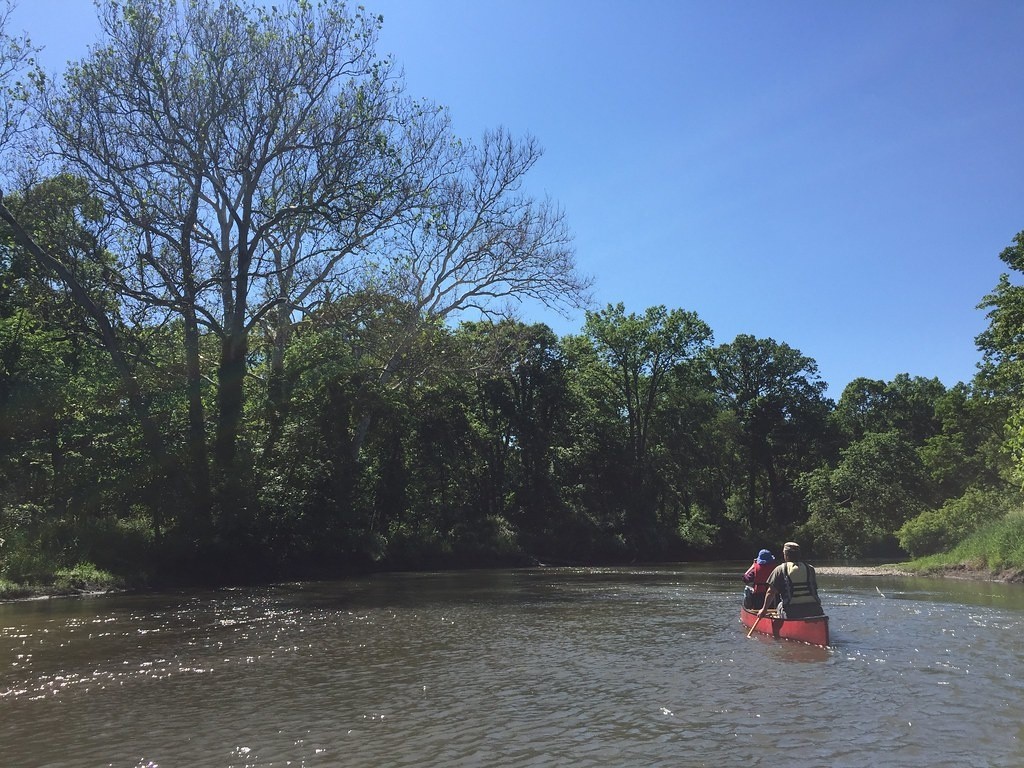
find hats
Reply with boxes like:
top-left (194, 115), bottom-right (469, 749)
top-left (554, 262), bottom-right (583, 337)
top-left (757, 549), bottom-right (775, 564)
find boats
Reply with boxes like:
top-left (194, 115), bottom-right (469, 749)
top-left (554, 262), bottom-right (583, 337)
top-left (742, 604), bottom-right (830, 646)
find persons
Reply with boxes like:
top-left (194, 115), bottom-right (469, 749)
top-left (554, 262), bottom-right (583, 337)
top-left (757, 542), bottom-right (823, 618)
top-left (743, 549), bottom-right (780, 610)
